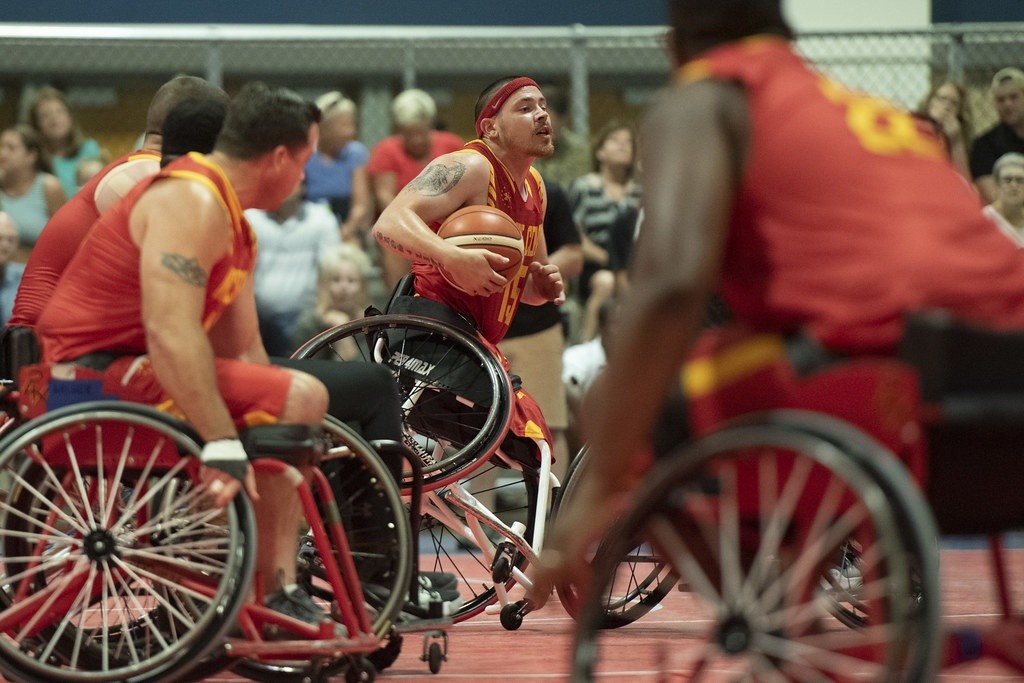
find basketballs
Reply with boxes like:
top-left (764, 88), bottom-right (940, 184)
top-left (435, 202), bottom-right (525, 296)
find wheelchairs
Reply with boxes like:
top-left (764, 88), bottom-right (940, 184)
top-left (282, 269), bottom-right (556, 629)
top-left (550, 443), bottom-right (686, 626)
top-left (0, 314), bottom-right (449, 683)
top-left (563, 306), bottom-right (1024, 683)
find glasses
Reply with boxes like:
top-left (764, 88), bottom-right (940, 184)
top-left (934, 92), bottom-right (962, 113)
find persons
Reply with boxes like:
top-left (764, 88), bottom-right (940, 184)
top-left (0, 1), bottom-right (1024, 639)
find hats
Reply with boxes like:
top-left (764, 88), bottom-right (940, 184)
top-left (992, 67), bottom-right (1024, 90)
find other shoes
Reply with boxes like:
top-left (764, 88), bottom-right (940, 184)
top-left (402, 571), bottom-right (463, 615)
top-left (264, 585), bottom-right (349, 641)
